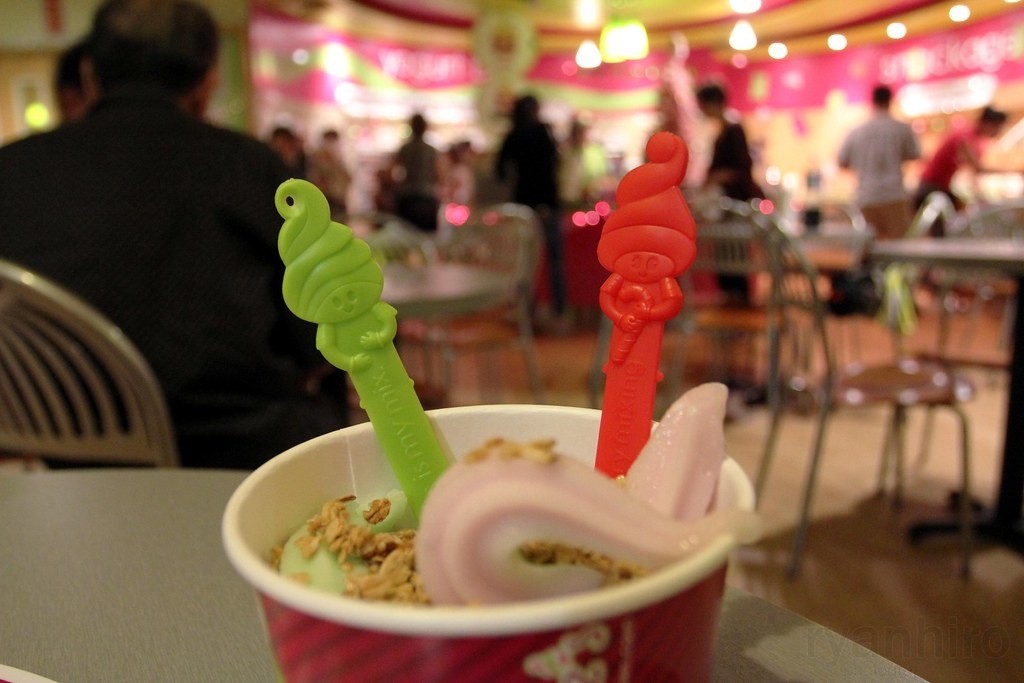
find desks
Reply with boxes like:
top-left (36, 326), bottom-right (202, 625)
top-left (860, 236), bottom-right (1023, 583)
top-left (371, 258), bottom-right (515, 322)
top-left (0, 469), bottom-right (930, 683)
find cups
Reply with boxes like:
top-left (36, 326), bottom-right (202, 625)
top-left (222, 404), bottom-right (759, 683)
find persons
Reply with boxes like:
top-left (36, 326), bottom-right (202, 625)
top-left (0, 0), bottom-right (352, 475)
top-left (837, 83), bottom-right (1008, 245)
top-left (377, 84), bottom-right (767, 330)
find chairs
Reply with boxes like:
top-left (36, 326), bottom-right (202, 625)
top-left (0, 261), bottom-right (178, 471)
top-left (342, 188), bottom-right (1023, 588)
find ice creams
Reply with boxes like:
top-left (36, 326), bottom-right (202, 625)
top-left (272, 382), bottom-right (730, 610)
